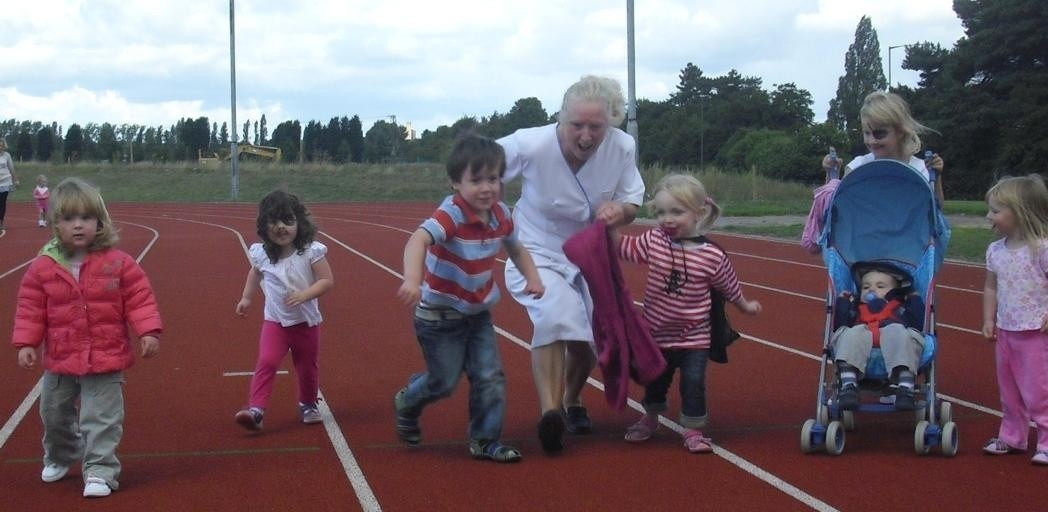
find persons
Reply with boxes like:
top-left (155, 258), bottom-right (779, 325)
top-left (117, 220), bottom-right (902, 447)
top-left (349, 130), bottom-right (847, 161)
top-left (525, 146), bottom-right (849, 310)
top-left (830, 261), bottom-right (928, 411)
top-left (12, 177), bottom-right (163, 500)
top-left (233, 190), bottom-right (335, 433)
top-left (488, 76), bottom-right (645, 455)
top-left (819, 88), bottom-right (949, 404)
top-left (0, 138), bottom-right (19, 238)
top-left (595, 172), bottom-right (765, 453)
top-left (33, 175), bottom-right (49, 228)
top-left (394, 134), bottom-right (545, 462)
top-left (981, 172), bottom-right (1048, 466)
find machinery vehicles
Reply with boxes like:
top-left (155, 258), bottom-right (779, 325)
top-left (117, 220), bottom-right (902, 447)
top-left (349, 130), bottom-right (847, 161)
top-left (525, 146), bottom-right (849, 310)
top-left (194, 139), bottom-right (283, 174)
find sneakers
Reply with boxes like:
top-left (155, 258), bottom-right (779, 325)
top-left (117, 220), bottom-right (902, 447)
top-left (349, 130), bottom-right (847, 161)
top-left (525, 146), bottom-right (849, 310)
top-left (42, 462), bottom-right (70, 482)
top-left (83, 476), bottom-right (111, 497)
top-left (298, 402), bottom-right (323, 423)
top-left (234, 408), bottom-right (263, 430)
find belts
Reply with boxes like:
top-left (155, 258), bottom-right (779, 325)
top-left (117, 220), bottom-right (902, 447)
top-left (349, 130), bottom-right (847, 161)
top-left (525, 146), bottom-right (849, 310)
top-left (414, 306), bottom-right (464, 321)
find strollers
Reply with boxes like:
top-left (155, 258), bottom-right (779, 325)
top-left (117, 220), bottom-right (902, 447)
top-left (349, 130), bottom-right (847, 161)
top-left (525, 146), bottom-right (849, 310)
top-left (796, 147), bottom-right (960, 456)
